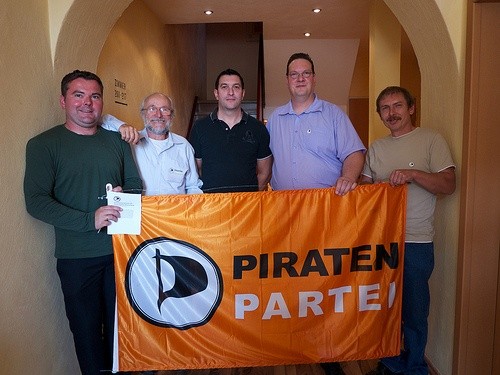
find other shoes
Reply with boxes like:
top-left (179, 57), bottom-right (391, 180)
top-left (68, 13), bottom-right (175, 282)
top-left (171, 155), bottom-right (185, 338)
top-left (318, 362), bottom-right (345, 375)
top-left (365, 361), bottom-right (396, 375)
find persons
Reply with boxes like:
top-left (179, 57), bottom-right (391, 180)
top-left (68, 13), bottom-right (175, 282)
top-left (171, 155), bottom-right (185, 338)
top-left (187, 70), bottom-right (274, 195)
top-left (24, 70), bottom-right (143, 375)
top-left (266, 53), bottom-right (368, 375)
top-left (100, 93), bottom-right (204, 195)
top-left (357, 87), bottom-right (456, 375)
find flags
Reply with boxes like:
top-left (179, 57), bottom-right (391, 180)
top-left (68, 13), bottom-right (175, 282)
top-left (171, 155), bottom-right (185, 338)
top-left (107, 181), bottom-right (408, 373)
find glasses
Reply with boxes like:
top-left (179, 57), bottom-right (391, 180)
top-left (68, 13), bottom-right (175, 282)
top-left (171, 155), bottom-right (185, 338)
top-left (143, 105), bottom-right (173, 115)
top-left (286, 69), bottom-right (314, 79)
